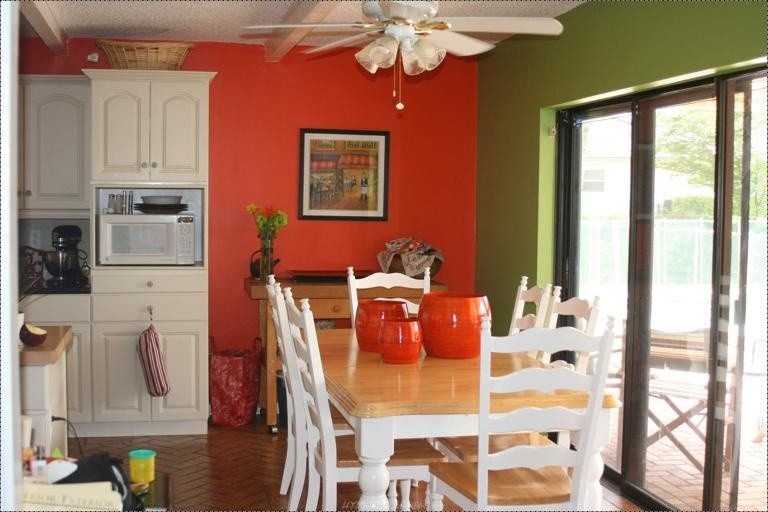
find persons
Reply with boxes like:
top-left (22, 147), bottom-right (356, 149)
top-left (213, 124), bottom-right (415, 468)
top-left (360, 171), bottom-right (368, 201)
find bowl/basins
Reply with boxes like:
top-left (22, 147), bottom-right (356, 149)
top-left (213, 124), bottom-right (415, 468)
top-left (141, 196), bottom-right (183, 204)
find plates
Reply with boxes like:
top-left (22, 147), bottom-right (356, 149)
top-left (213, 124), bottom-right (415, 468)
top-left (133, 203), bottom-right (189, 214)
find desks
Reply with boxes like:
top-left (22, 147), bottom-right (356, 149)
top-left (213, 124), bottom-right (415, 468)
top-left (245, 277), bottom-right (449, 434)
top-left (121, 469), bottom-right (172, 512)
top-left (20, 325), bottom-right (73, 457)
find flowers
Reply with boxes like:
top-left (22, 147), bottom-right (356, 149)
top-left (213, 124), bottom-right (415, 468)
top-left (246, 203), bottom-right (289, 238)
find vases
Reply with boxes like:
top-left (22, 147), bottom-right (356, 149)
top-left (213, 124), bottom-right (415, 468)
top-left (259, 238), bottom-right (271, 280)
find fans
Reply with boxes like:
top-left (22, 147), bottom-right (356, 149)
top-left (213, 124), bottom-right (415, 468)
top-left (239, 1), bottom-right (564, 57)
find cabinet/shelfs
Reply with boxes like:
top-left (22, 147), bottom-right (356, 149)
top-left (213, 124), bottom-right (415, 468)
top-left (18, 74), bottom-right (92, 218)
top-left (19, 267), bottom-right (209, 437)
top-left (81, 69), bottom-right (218, 189)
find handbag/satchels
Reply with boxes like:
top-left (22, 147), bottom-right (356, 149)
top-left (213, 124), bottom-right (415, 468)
top-left (208, 336), bottom-right (262, 428)
top-left (51, 454), bottom-right (144, 511)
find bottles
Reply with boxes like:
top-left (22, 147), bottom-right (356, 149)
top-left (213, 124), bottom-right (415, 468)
top-left (107, 194), bottom-right (116, 214)
top-left (128, 449), bottom-right (157, 484)
top-left (80, 262), bottom-right (91, 280)
top-left (116, 194), bottom-right (123, 213)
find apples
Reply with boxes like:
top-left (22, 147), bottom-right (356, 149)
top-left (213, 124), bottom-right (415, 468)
top-left (19, 324), bottom-right (48, 346)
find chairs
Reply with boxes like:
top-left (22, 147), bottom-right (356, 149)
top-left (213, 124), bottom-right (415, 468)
top-left (432, 285), bottom-right (601, 462)
top-left (283, 287), bottom-right (449, 512)
top-left (587, 328), bottom-right (768, 476)
top-left (266, 274), bottom-right (355, 496)
top-left (507, 275), bottom-right (552, 360)
top-left (346, 265), bottom-right (431, 328)
top-left (429, 316), bottom-right (616, 512)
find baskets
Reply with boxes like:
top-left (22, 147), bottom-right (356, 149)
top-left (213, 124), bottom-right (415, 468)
top-left (95, 39), bottom-right (195, 70)
top-left (390, 254), bottom-right (442, 279)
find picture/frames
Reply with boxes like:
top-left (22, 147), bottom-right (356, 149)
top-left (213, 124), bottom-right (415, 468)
top-left (298, 128), bottom-right (390, 222)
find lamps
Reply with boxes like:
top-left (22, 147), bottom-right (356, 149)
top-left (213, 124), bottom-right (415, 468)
top-left (354, 27), bottom-right (447, 110)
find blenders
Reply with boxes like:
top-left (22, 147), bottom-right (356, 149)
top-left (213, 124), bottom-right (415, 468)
top-left (43, 224), bottom-right (89, 288)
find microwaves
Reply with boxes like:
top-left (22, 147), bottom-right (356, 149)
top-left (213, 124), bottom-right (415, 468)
top-left (99, 211), bottom-right (195, 266)
